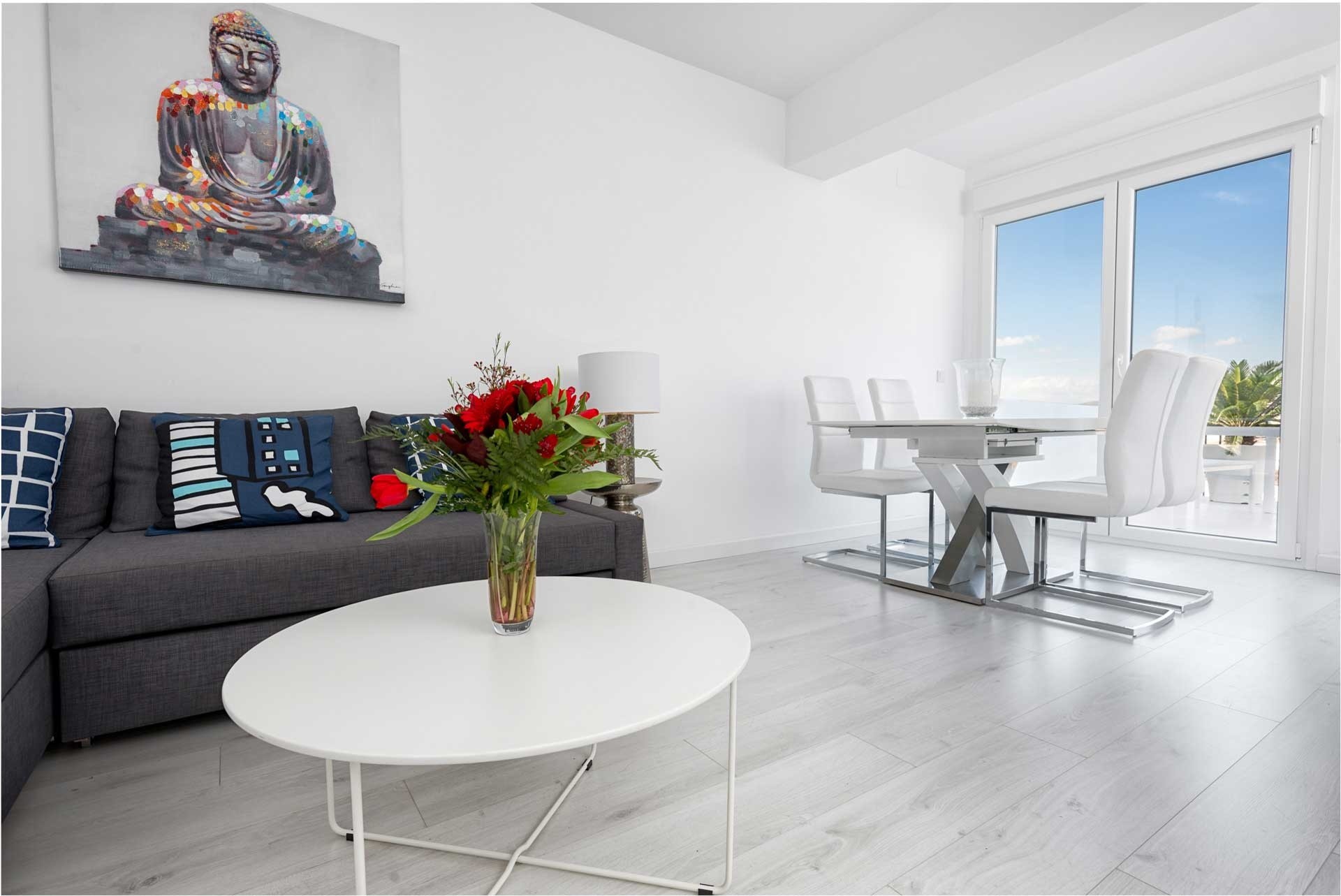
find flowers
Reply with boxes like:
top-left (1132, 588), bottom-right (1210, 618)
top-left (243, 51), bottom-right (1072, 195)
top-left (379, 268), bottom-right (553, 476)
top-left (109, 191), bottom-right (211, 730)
top-left (350, 333), bottom-right (663, 620)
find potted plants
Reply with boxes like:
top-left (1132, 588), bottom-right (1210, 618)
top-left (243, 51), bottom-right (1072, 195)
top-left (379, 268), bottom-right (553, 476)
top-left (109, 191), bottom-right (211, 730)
top-left (1203, 359), bottom-right (1283, 503)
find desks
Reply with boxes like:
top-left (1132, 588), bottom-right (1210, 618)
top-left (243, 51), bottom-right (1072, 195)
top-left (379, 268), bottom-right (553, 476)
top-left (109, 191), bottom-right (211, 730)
top-left (809, 418), bottom-right (1110, 607)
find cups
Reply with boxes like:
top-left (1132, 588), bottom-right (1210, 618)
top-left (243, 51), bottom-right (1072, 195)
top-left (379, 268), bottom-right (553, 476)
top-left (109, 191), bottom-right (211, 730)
top-left (953, 357), bottom-right (1006, 418)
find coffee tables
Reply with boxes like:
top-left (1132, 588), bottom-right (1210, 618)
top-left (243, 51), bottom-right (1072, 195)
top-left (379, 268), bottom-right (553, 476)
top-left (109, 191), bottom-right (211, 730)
top-left (225, 575), bottom-right (754, 895)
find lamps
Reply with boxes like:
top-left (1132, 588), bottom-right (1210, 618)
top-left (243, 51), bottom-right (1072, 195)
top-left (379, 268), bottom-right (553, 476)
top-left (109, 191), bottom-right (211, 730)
top-left (578, 350), bottom-right (663, 585)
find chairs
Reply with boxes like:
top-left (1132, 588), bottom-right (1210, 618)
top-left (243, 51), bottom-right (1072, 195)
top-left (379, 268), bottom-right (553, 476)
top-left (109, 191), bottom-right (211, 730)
top-left (1040, 354), bottom-right (1229, 616)
top-left (984, 349), bottom-right (1192, 638)
top-left (803, 374), bottom-right (934, 581)
top-left (867, 379), bottom-right (949, 564)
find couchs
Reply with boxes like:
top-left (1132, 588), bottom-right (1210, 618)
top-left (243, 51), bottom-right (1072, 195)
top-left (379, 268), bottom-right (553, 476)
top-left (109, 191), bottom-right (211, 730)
top-left (2, 404), bottom-right (649, 824)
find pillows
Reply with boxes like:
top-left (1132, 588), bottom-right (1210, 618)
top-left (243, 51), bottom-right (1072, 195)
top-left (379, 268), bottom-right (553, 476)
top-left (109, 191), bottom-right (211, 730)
top-left (142, 410), bottom-right (350, 537)
top-left (388, 414), bottom-right (558, 513)
top-left (1, 407), bottom-right (75, 550)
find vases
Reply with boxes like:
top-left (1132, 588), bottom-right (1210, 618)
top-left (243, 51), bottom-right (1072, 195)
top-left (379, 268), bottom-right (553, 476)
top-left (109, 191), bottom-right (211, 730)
top-left (476, 507), bottom-right (543, 636)
